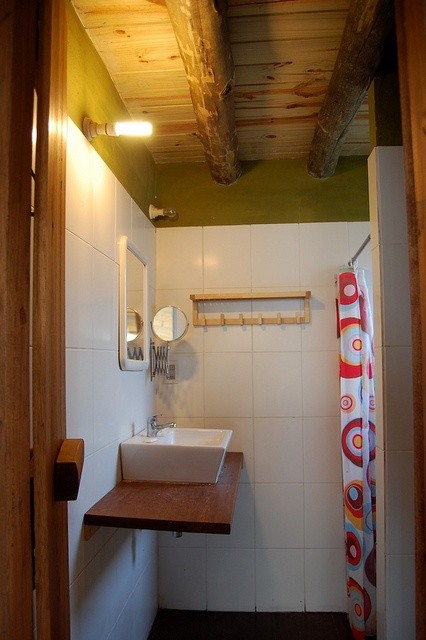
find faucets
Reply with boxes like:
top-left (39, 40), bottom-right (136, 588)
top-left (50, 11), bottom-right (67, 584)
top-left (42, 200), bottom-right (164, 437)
top-left (147, 414), bottom-right (177, 437)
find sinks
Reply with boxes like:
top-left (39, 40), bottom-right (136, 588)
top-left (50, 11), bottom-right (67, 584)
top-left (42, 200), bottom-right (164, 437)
top-left (121, 427), bottom-right (233, 483)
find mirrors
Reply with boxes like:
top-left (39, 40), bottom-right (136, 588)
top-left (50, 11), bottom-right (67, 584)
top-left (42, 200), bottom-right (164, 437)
top-left (151, 306), bottom-right (189, 343)
top-left (119, 236), bottom-right (151, 370)
top-left (126, 308), bottom-right (143, 341)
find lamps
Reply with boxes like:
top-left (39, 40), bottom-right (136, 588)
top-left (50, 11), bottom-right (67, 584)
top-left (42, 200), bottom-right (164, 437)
top-left (149, 205), bottom-right (175, 220)
top-left (83, 118), bottom-right (152, 143)
